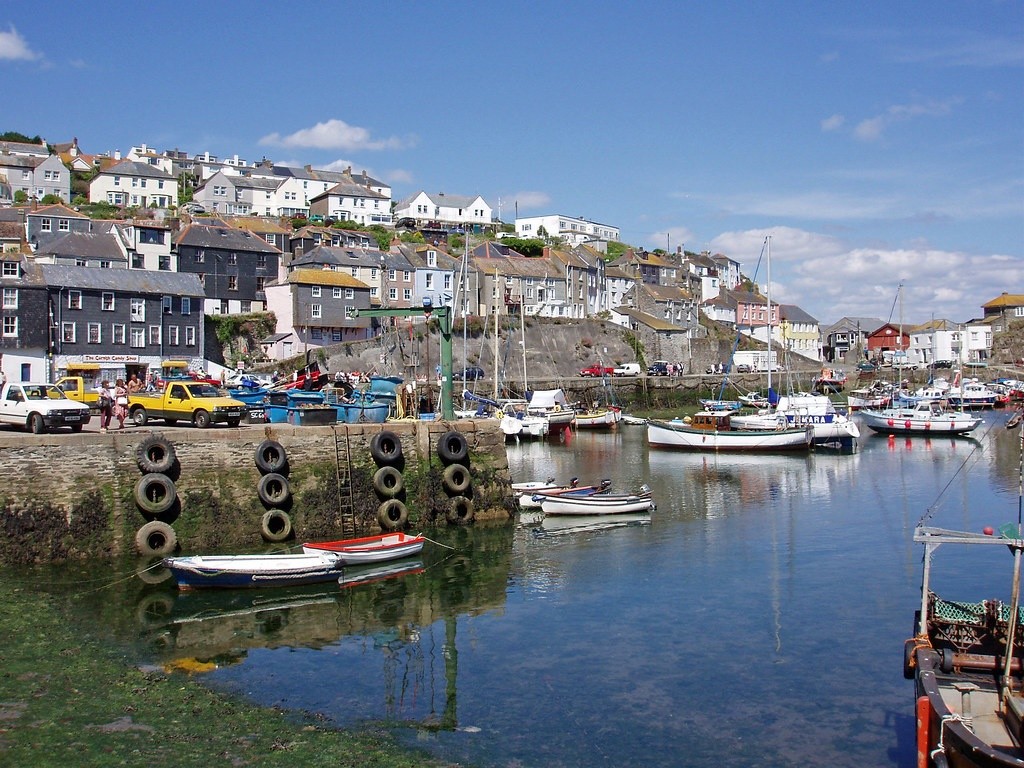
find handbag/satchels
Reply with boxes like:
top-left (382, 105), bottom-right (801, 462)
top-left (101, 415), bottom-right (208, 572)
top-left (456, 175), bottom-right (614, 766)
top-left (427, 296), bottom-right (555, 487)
top-left (117, 397), bottom-right (128, 406)
top-left (96, 397), bottom-right (109, 408)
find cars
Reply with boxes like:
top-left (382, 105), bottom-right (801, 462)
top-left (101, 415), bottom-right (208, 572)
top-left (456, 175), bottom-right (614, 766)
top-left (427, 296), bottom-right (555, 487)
top-left (452, 366), bottom-right (485, 380)
top-left (737, 364), bottom-right (751, 373)
top-left (706, 363), bottom-right (727, 374)
top-left (188, 372), bottom-right (271, 390)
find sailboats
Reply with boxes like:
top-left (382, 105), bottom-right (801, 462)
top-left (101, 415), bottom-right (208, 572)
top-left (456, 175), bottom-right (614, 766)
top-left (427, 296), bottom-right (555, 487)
top-left (847, 277), bottom-right (1024, 433)
top-left (903, 377), bottom-right (1024, 768)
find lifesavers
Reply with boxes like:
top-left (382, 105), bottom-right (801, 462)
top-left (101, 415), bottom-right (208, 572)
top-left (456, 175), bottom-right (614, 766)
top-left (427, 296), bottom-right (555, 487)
top-left (913, 610), bottom-right (921, 637)
top-left (143, 630), bottom-right (175, 654)
top-left (440, 526), bottom-right (474, 612)
top-left (135, 520), bottom-right (179, 556)
top-left (136, 554), bottom-right (173, 584)
top-left (254, 439), bottom-right (286, 472)
top-left (496, 410), bottom-right (503, 419)
top-left (373, 466), bottom-right (404, 498)
top-left (257, 473), bottom-right (290, 506)
top-left (133, 472), bottom-right (177, 514)
top-left (554, 405), bottom-right (561, 412)
top-left (256, 611), bottom-right (287, 637)
top-left (437, 431), bottom-right (475, 525)
top-left (954, 373), bottom-right (963, 387)
top-left (136, 435), bottom-right (176, 472)
top-left (137, 592), bottom-right (175, 629)
top-left (904, 641), bottom-right (916, 679)
top-left (264, 543), bottom-right (291, 554)
top-left (378, 499), bottom-right (407, 529)
top-left (370, 430), bottom-right (402, 463)
top-left (259, 509), bottom-right (292, 541)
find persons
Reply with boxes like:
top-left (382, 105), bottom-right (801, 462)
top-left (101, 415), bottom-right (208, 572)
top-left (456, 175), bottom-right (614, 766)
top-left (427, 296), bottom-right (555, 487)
top-left (221, 369), bottom-right (226, 386)
top-left (198, 367), bottom-right (204, 374)
top-left (146, 372), bottom-right (156, 391)
top-left (436, 363), bottom-right (441, 380)
top-left (711, 362), bottom-right (723, 375)
top-left (227, 370), bottom-right (231, 378)
top-left (0, 371), bottom-right (7, 399)
top-left (114, 379), bottom-right (127, 430)
top-left (98, 381), bottom-right (113, 431)
top-left (666, 362), bottom-right (684, 376)
top-left (334, 370), bottom-right (378, 385)
top-left (128, 374), bottom-right (145, 393)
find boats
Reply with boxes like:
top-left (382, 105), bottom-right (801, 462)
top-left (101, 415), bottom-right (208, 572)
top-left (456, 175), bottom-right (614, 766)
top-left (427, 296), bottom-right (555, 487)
top-left (811, 364), bottom-right (848, 395)
top-left (302, 532), bottom-right (426, 565)
top-left (162, 553), bottom-right (342, 587)
top-left (229, 231), bottom-right (860, 451)
top-left (511, 475), bottom-right (657, 515)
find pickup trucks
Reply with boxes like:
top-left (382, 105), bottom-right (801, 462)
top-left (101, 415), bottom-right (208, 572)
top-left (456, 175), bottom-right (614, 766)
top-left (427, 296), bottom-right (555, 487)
top-left (0, 376), bottom-right (99, 434)
top-left (646, 360), bottom-right (678, 376)
top-left (579, 364), bottom-right (614, 377)
top-left (128, 382), bottom-right (248, 429)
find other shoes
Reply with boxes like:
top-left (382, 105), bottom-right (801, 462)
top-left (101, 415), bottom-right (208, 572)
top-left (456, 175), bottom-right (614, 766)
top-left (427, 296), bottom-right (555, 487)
top-left (100, 427), bottom-right (108, 431)
top-left (119, 425), bottom-right (124, 429)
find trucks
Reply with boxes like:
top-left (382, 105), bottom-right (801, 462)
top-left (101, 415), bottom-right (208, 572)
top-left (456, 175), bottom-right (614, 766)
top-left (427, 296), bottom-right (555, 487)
top-left (614, 363), bottom-right (641, 377)
